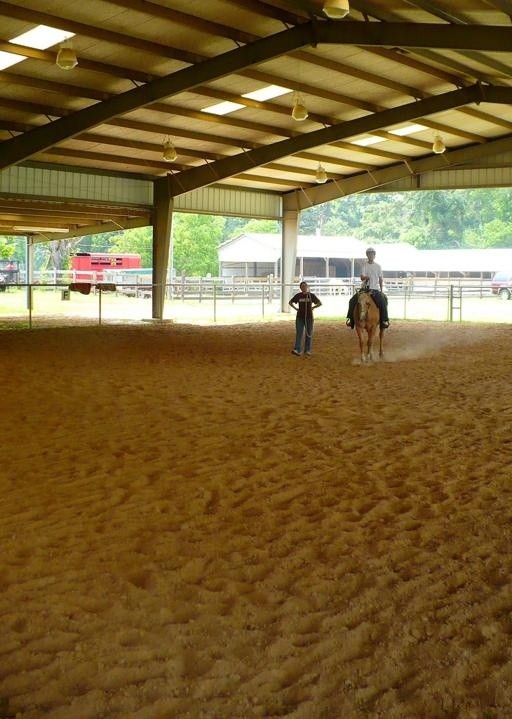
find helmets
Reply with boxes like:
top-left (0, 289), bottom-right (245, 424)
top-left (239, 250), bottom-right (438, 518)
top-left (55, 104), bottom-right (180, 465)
top-left (366, 248), bottom-right (376, 254)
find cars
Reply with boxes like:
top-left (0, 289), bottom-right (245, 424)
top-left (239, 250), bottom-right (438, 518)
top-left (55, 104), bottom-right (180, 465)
top-left (306, 276), bottom-right (362, 295)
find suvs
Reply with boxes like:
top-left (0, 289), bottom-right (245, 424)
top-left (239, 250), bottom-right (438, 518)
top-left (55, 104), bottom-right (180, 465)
top-left (491, 271), bottom-right (512, 299)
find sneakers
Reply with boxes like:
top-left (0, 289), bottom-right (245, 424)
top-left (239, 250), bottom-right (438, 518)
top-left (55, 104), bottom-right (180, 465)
top-left (304, 352), bottom-right (311, 356)
top-left (347, 319), bottom-right (352, 326)
top-left (383, 321), bottom-right (389, 325)
top-left (292, 350), bottom-right (301, 356)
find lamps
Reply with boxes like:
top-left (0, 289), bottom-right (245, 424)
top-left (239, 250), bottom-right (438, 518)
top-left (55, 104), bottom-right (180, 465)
top-left (321, 0), bottom-right (350, 18)
top-left (432, 130), bottom-right (446, 154)
top-left (292, 89), bottom-right (309, 121)
top-left (315, 161), bottom-right (328, 184)
top-left (55, 33), bottom-right (79, 70)
top-left (161, 136), bottom-right (178, 161)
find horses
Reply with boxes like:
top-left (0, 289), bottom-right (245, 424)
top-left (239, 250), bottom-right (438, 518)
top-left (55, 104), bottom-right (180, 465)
top-left (353, 288), bottom-right (388, 362)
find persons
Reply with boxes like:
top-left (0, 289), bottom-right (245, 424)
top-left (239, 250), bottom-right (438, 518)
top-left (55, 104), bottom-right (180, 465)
top-left (346, 248), bottom-right (389, 327)
top-left (8, 261), bottom-right (15, 282)
top-left (289, 282), bottom-right (322, 356)
